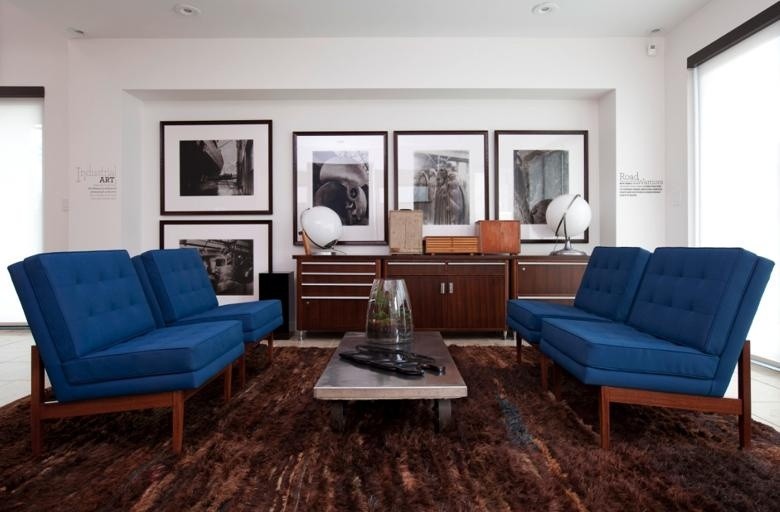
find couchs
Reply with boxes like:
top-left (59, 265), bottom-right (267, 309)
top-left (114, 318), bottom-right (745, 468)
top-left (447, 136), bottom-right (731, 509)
top-left (505, 247), bottom-right (653, 394)
top-left (132, 247), bottom-right (280, 393)
top-left (5, 251), bottom-right (246, 453)
top-left (540, 246), bottom-right (773, 453)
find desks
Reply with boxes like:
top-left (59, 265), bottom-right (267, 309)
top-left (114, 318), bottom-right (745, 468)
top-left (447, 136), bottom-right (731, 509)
top-left (312, 330), bottom-right (468, 435)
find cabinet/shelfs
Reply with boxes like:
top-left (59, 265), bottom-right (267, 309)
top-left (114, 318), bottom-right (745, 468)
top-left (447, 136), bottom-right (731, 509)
top-left (298, 255), bottom-right (384, 340)
top-left (514, 254), bottom-right (594, 307)
top-left (382, 253), bottom-right (511, 341)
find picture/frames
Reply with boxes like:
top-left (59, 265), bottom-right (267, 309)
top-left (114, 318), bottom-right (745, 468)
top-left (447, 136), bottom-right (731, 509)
top-left (392, 130), bottom-right (490, 249)
top-left (158, 120), bottom-right (274, 216)
top-left (291, 131), bottom-right (389, 246)
top-left (159, 219), bottom-right (271, 306)
top-left (495, 130), bottom-right (588, 245)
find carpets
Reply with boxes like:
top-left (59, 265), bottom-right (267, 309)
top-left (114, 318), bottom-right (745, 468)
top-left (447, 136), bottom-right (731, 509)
top-left (0, 344), bottom-right (778, 511)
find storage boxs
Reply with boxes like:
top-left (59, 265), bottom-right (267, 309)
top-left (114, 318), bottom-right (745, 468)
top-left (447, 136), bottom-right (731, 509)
top-left (476, 220), bottom-right (521, 255)
